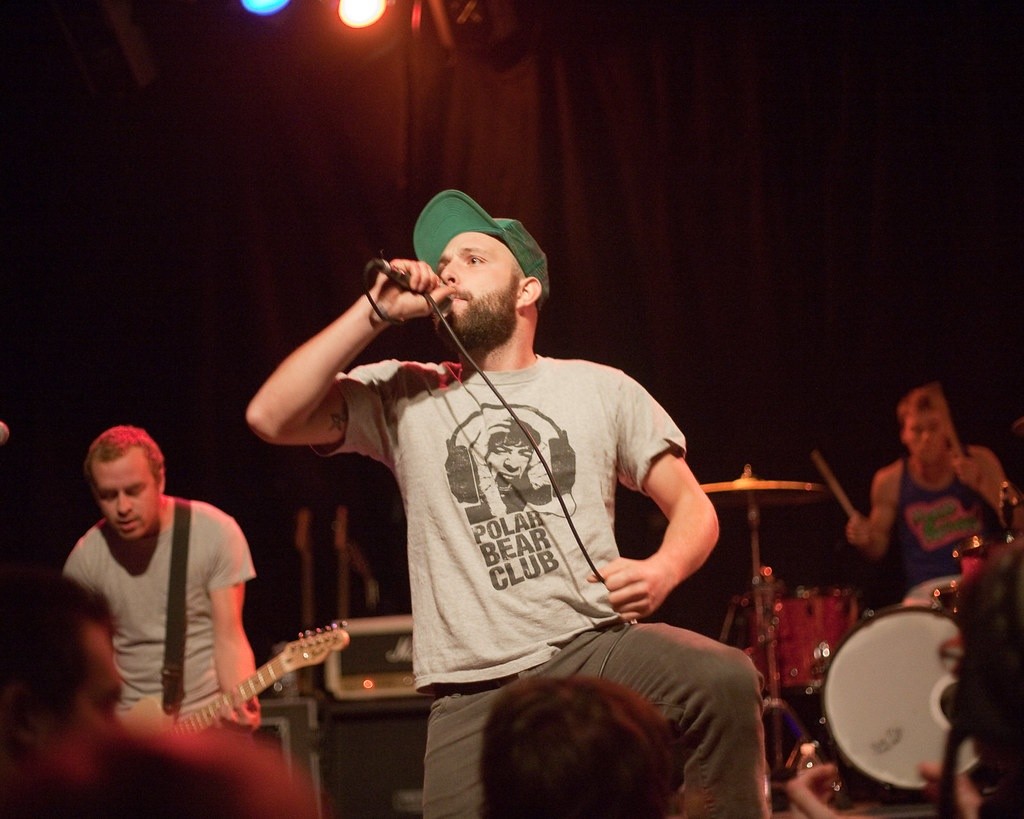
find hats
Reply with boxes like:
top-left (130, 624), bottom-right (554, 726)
top-left (413, 189), bottom-right (550, 311)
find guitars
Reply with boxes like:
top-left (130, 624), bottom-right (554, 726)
top-left (117, 617), bottom-right (350, 745)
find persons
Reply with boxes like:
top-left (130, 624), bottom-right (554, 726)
top-left (482, 676), bottom-right (681, 819)
top-left (245, 188), bottom-right (771, 819)
top-left (0, 561), bottom-right (323, 818)
top-left (63, 425), bottom-right (262, 735)
top-left (846, 386), bottom-right (1021, 592)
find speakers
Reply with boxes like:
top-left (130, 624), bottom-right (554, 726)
top-left (319, 698), bottom-right (435, 819)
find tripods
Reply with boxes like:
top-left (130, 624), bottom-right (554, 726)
top-left (744, 488), bottom-right (824, 781)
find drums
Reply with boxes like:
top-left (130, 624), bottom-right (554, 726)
top-left (748, 585), bottom-right (862, 692)
top-left (902, 573), bottom-right (965, 610)
top-left (817, 602), bottom-right (984, 792)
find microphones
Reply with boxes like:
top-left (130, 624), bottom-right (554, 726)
top-left (998, 481), bottom-right (1014, 530)
top-left (374, 257), bottom-right (454, 311)
top-left (0, 421), bottom-right (9, 446)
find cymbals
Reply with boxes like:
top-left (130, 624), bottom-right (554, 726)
top-left (954, 524), bottom-right (1024, 561)
top-left (694, 474), bottom-right (828, 507)
